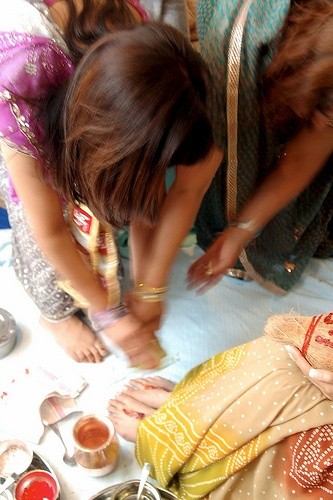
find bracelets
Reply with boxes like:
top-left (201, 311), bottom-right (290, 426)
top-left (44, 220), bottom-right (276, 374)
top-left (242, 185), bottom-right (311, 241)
top-left (129, 280), bottom-right (169, 303)
top-left (229, 214), bottom-right (262, 240)
top-left (87, 303), bottom-right (130, 332)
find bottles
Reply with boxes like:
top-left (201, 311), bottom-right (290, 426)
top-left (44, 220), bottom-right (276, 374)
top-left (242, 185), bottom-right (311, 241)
top-left (72, 414), bottom-right (118, 477)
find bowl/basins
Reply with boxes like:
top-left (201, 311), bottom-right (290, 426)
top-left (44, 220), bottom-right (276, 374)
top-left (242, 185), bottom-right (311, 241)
top-left (0, 439), bottom-right (33, 476)
top-left (112, 481), bottom-right (160, 500)
top-left (14, 470), bottom-right (60, 500)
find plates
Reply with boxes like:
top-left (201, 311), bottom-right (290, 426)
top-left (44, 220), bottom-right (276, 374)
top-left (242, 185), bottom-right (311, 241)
top-left (89, 483), bottom-right (178, 500)
top-left (0, 451), bottom-right (61, 500)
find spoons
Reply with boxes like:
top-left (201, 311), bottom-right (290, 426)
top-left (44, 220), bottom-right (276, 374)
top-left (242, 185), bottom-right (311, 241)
top-left (50, 424), bottom-right (76, 466)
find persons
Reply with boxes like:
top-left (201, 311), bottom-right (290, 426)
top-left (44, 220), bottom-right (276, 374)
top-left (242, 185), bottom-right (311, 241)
top-left (0, 0), bottom-right (225, 368)
top-left (106, 311), bottom-right (332, 500)
top-left (185, 0), bottom-right (332, 297)
